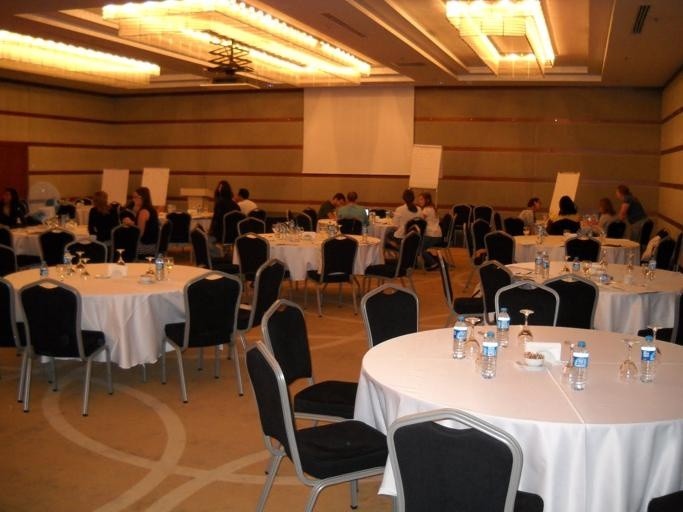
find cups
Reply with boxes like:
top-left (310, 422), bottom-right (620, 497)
top-left (145, 256), bottom-right (158, 280)
top-left (56, 263), bottom-right (66, 281)
top-left (646, 324), bottom-right (663, 364)
top-left (560, 340), bottom-right (578, 385)
top-left (65, 254), bottom-right (76, 280)
top-left (641, 264), bottom-right (649, 287)
top-left (582, 260), bottom-right (593, 281)
top-left (116, 248), bottom-right (127, 265)
top-left (474, 330), bottom-right (488, 370)
top-left (139, 274), bottom-right (152, 284)
top-left (463, 317), bottom-right (481, 361)
top-left (79, 258), bottom-right (91, 282)
top-left (517, 309), bottom-right (535, 350)
top-left (560, 255), bottom-right (571, 276)
top-left (523, 227), bottom-right (530, 242)
top-left (618, 339), bottom-right (641, 382)
top-left (75, 251), bottom-right (85, 273)
top-left (303, 234), bottom-right (312, 240)
top-left (164, 257), bottom-right (175, 278)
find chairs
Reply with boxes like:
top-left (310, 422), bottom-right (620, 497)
top-left (236, 217), bottom-right (266, 234)
top-left (165, 210), bottom-right (191, 244)
top-left (473, 220), bottom-right (492, 256)
top-left (369, 208), bottom-right (388, 217)
top-left (63, 236), bottom-right (108, 265)
top-left (190, 228), bottom-right (245, 292)
top-left (0, 244), bottom-right (18, 279)
top-left (247, 207), bottom-right (267, 219)
top-left (478, 260), bottom-right (514, 323)
top-left (214, 210), bottom-right (246, 257)
top-left (19, 216), bottom-right (41, 226)
top-left (504, 218), bottom-right (524, 236)
top-left (198, 258), bottom-right (286, 372)
top-left (385, 224), bottom-right (420, 272)
top-left (452, 204), bottom-right (471, 247)
top-left (196, 224), bottom-right (231, 263)
top-left (0, 225), bottom-right (15, 249)
top-left (303, 234), bottom-right (360, 318)
top-left (473, 203), bottom-right (492, 227)
top-left (18, 277), bottom-right (113, 417)
top-left (261, 299), bottom-right (359, 476)
top-left (362, 231), bottom-right (418, 294)
top-left (647, 491), bottom-right (683, 512)
top-left (0, 275), bottom-right (52, 402)
top-left (360, 283), bottom-right (420, 349)
top-left (433, 212), bottom-right (454, 267)
top-left (640, 227), bottom-right (671, 265)
top-left (162, 271), bottom-right (247, 405)
top-left (39, 226), bottom-right (76, 267)
top-left (245, 341), bottom-right (389, 511)
top-left (111, 223), bottom-right (141, 262)
top-left (110, 201), bottom-right (120, 221)
top-left (494, 279), bottom-right (560, 327)
top-left (484, 230), bottom-right (516, 265)
top-left (74, 199), bottom-right (90, 205)
top-left (137, 221), bottom-right (170, 260)
top-left (235, 232), bottom-right (293, 301)
top-left (607, 219), bottom-right (626, 238)
top-left (564, 235), bottom-right (602, 263)
top-left (337, 218), bottom-right (363, 236)
top-left (638, 294), bottom-right (683, 346)
top-left (655, 237), bottom-right (676, 270)
top-left (436, 250), bottom-right (484, 327)
top-left (541, 274), bottom-right (600, 329)
top-left (671, 233), bottom-right (683, 265)
top-left (56, 204), bottom-right (75, 225)
top-left (386, 407), bottom-right (544, 511)
top-left (289, 212), bottom-right (313, 232)
top-left (469, 205), bottom-right (475, 210)
top-left (546, 217), bottom-right (580, 236)
top-left (465, 219), bottom-right (485, 290)
top-left (639, 220), bottom-right (655, 250)
top-left (382, 216), bottom-right (427, 254)
top-left (494, 212), bottom-right (502, 230)
top-left (304, 206), bottom-right (316, 231)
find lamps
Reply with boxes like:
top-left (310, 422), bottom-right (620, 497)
top-left (0, 27), bottom-right (160, 90)
top-left (444, 0), bottom-right (556, 79)
top-left (101, 1), bottom-right (372, 86)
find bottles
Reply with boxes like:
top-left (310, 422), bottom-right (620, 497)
top-left (452, 316), bottom-right (467, 359)
top-left (649, 257), bottom-right (656, 270)
top-left (362, 224), bottom-right (368, 242)
top-left (601, 270), bottom-right (608, 289)
top-left (480, 331), bottom-right (497, 379)
top-left (156, 253), bottom-right (164, 281)
top-left (641, 336), bottom-right (655, 384)
top-left (497, 308), bottom-right (510, 348)
top-left (571, 341), bottom-right (588, 391)
top-left (572, 257), bottom-right (580, 281)
top-left (39, 261), bottom-right (48, 287)
top-left (535, 251), bottom-right (542, 274)
top-left (64, 250), bottom-right (71, 276)
top-left (542, 255), bottom-right (550, 280)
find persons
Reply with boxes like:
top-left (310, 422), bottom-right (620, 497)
top-left (88, 189), bottom-right (121, 250)
top-left (336, 191), bottom-right (370, 235)
top-left (594, 198), bottom-right (616, 238)
top-left (212, 181), bottom-right (234, 198)
top-left (0, 188), bottom-right (27, 233)
top-left (316, 192), bottom-right (345, 222)
top-left (122, 186), bottom-right (159, 255)
top-left (386, 189), bottom-right (421, 251)
top-left (403, 193), bottom-right (444, 270)
top-left (205, 189), bottom-right (241, 244)
top-left (235, 188), bottom-right (258, 217)
top-left (609, 184), bottom-right (649, 244)
top-left (518, 197), bottom-right (542, 230)
top-left (545, 196), bottom-right (580, 235)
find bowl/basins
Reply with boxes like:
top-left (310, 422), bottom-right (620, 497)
top-left (522, 357), bottom-right (546, 366)
